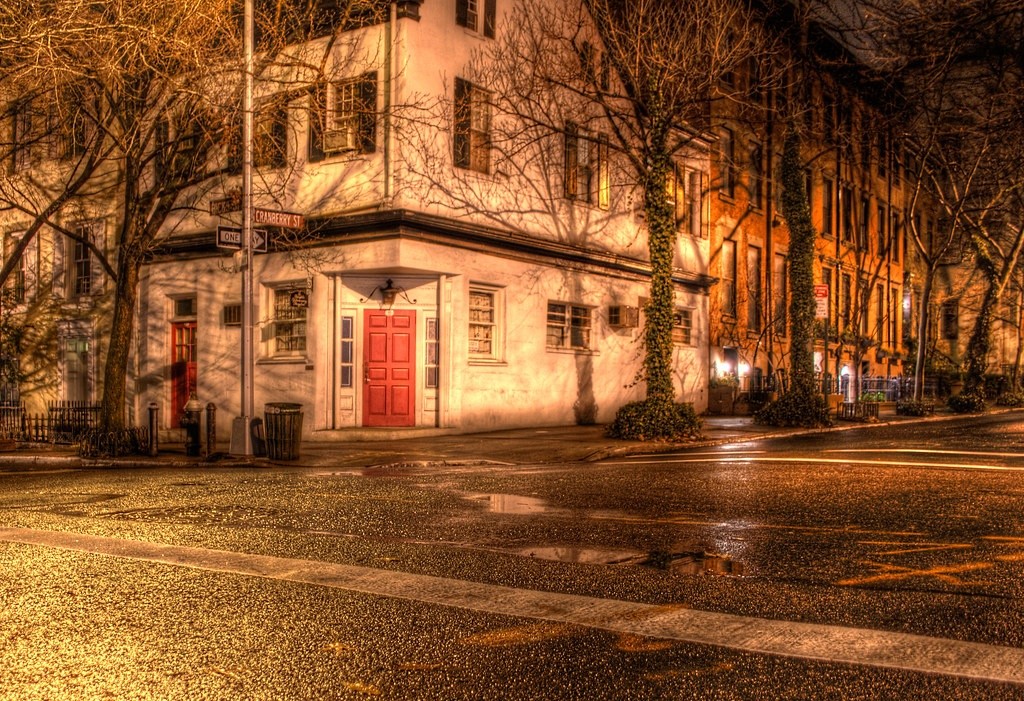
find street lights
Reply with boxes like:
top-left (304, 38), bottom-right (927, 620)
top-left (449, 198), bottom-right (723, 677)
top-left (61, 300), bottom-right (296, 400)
top-left (821, 58), bottom-right (853, 394)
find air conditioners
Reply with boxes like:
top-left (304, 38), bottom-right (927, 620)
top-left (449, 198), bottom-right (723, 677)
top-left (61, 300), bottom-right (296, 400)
top-left (609, 305), bottom-right (639, 328)
top-left (319, 127), bottom-right (358, 153)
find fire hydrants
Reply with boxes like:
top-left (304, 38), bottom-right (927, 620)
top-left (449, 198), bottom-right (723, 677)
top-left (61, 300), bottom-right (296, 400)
top-left (180, 390), bottom-right (202, 456)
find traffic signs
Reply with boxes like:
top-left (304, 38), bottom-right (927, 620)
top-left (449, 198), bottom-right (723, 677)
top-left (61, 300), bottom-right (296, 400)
top-left (216, 224), bottom-right (271, 253)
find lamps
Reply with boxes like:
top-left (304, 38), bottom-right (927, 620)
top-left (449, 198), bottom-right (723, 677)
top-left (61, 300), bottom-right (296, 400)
top-left (359, 278), bottom-right (417, 317)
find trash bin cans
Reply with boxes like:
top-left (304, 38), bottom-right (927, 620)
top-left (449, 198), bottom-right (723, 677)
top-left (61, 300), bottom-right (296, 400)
top-left (264, 402), bottom-right (304, 461)
